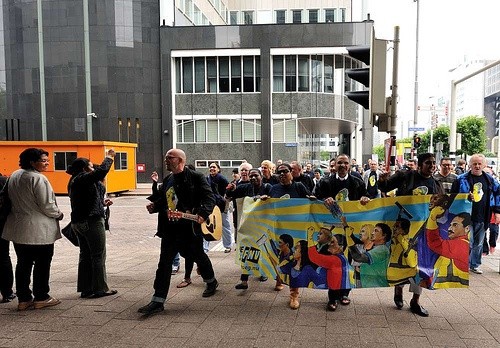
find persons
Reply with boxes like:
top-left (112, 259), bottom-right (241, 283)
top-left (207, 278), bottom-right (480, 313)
top-left (377, 153), bottom-right (474, 317)
top-left (314, 154), bottom-right (370, 310)
top-left (226, 153), bottom-right (500, 281)
top-left (259, 163), bottom-right (317, 309)
top-left (200, 162), bottom-right (230, 253)
top-left (227, 167), bottom-right (274, 289)
top-left (137, 148), bottom-right (218, 312)
top-left (66, 147), bottom-right (117, 297)
top-left (0, 172), bottom-right (16, 303)
top-left (8, 148), bottom-right (63, 311)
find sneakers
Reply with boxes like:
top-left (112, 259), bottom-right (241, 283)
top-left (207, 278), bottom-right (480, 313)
top-left (425, 269), bottom-right (438, 290)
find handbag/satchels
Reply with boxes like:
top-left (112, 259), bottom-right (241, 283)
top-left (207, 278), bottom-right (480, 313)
top-left (207, 175), bottom-right (226, 213)
top-left (61, 222), bottom-right (80, 247)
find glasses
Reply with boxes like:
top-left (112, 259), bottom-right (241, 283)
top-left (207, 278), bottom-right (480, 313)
top-left (441, 163), bottom-right (450, 166)
top-left (277, 169), bottom-right (290, 175)
top-left (165, 156), bottom-right (179, 160)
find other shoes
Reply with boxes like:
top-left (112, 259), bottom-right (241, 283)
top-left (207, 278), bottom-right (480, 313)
top-left (172, 264), bottom-right (179, 275)
top-left (235, 284), bottom-right (248, 290)
top-left (177, 279), bottom-right (192, 288)
top-left (202, 277), bottom-right (219, 297)
top-left (224, 248), bottom-right (231, 253)
top-left (471, 268), bottom-right (483, 274)
top-left (340, 295), bottom-right (350, 305)
top-left (137, 301), bottom-right (165, 315)
top-left (81, 290), bottom-right (117, 299)
top-left (18, 298), bottom-right (34, 310)
top-left (260, 277), bottom-right (268, 281)
top-left (1, 293), bottom-right (16, 303)
top-left (327, 299), bottom-right (337, 312)
top-left (276, 285), bottom-right (283, 291)
top-left (33, 297), bottom-right (61, 309)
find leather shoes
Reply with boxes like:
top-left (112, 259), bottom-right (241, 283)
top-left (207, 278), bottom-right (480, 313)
top-left (409, 299), bottom-right (429, 316)
top-left (394, 287), bottom-right (404, 309)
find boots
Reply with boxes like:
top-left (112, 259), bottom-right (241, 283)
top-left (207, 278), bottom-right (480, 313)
top-left (289, 287), bottom-right (299, 309)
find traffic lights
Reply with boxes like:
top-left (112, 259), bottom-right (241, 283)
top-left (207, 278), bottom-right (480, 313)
top-left (344, 26), bottom-right (386, 126)
top-left (414, 137), bottom-right (421, 148)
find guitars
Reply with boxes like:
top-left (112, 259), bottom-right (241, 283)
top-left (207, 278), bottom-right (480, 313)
top-left (167, 204), bottom-right (223, 241)
